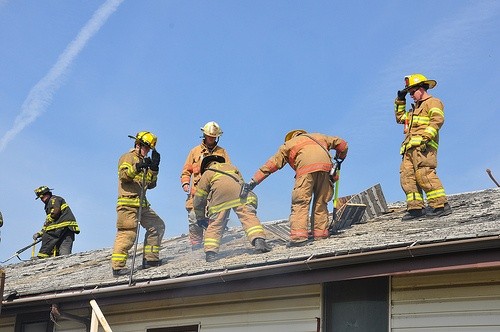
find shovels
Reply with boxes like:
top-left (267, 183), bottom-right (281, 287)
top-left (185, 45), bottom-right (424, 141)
top-left (327, 157), bottom-right (342, 232)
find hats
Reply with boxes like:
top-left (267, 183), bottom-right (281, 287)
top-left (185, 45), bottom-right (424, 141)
top-left (34, 186), bottom-right (54, 200)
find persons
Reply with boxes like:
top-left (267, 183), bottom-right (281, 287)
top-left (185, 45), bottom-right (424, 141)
top-left (240, 129), bottom-right (348, 248)
top-left (394, 74), bottom-right (452, 221)
top-left (193, 155), bottom-right (272, 261)
top-left (181, 121), bottom-right (230, 249)
top-left (112, 132), bottom-right (165, 277)
top-left (30, 185), bottom-right (80, 260)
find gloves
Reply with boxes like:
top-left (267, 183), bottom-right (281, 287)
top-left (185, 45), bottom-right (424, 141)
top-left (136, 157), bottom-right (151, 173)
top-left (419, 143), bottom-right (428, 152)
top-left (198, 218), bottom-right (208, 229)
top-left (248, 179), bottom-right (256, 191)
top-left (32, 233), bottom-right (40, 241)
top-left (150, 151), bottom-right (160, 172)
top-left (397, 89), bottom-right (408, 101)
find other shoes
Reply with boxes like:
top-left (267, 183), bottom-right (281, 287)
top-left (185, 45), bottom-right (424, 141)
top-left (286, 241), bottom-right (308, 247)
top-left (402, 209), bottom-right (426, 220)
top-left (254, 238), bottom-right (272, 253)
top-left (206, 251), bottom-right (216, 261)
top-left (142, 258), bottom-right (162, 268)
top-left (426, 203), bottom-right (452, 218)
top-left (112, 268), bottom-right (136, 276)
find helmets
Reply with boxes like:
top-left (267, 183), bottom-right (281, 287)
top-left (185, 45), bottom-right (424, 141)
top-left (202, 120), bottom-right (222, 138)
top-left (284, 130), bottom-right (306, 141)
top-left (401, 74), bottom-right (437, 89)
top-left (200, 155), bottom-right (225, 175)
top-left (136, 131), bottom-right (157, 148)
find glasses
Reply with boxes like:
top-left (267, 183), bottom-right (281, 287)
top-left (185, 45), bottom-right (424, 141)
top-left (410, 87), bottom-right (420, 95)
